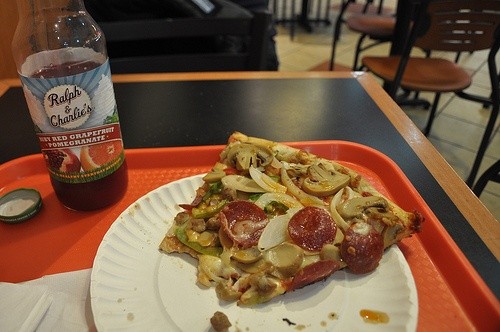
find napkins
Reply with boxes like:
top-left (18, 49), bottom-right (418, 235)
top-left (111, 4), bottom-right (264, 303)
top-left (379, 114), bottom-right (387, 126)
top-left (18, 267), bottom-right (97, 332)
top-left (0, 282), bottom-right (53, 332)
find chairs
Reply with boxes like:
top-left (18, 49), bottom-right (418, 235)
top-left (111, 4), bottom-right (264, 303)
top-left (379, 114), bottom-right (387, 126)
top-left (329, 0), bottom-right (396, 71)
top-left (98, 12), bottom-right (272, 74)
top-left (359, 0), bottom-right (500, 138)
top-left (465, 28), bottom-right (500, 198)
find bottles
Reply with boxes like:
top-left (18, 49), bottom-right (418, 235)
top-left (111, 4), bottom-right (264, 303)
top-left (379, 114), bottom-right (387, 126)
top-left (12, 0), bottom-right (129, 212)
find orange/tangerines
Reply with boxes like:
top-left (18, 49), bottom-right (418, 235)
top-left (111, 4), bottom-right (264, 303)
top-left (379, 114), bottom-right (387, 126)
top-left (80, 140), bottom-right (122, 171)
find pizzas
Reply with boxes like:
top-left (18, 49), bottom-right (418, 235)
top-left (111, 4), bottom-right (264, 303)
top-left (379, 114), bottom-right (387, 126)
top-left (159, 131), bottom-right (425, 306)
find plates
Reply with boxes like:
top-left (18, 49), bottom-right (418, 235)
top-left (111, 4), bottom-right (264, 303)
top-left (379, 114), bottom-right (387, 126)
top-left (90, 171), bottom-right (418, 332)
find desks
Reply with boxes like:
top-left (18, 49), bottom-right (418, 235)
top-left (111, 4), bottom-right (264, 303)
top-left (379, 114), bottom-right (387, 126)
top-left (0, 71), bottom-right (500, 300)
